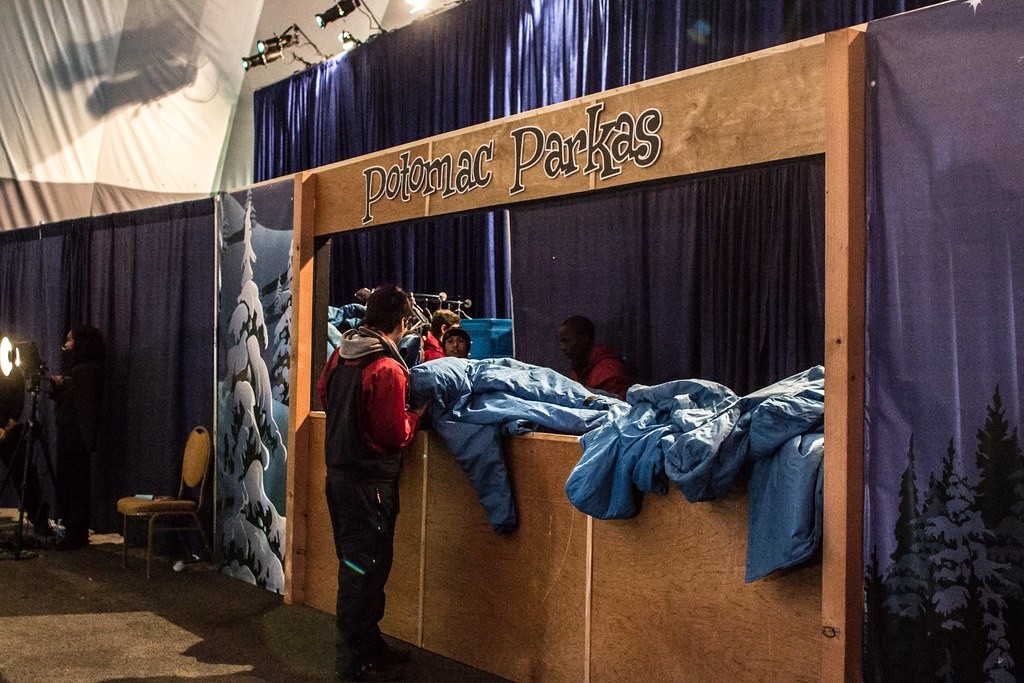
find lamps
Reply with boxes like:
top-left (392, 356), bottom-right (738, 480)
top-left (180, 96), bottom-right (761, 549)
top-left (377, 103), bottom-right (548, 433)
top-left (256, 35), bottom-right (293, 54)
top-left (242, 52), bottom-right (284, 71)
top-left (314, 0), bottom-right (362, 29)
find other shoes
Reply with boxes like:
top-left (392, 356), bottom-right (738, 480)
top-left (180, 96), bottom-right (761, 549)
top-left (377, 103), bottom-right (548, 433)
top-left (55, 533), bottom-right (89, 550)
top-left (336, 659), bottom-right (403, 683)
top-left (353, 634), bottom-right (411, 663)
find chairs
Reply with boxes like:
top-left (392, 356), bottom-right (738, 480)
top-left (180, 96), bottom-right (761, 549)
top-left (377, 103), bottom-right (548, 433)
top-left (117, 425), bottom-right (213, 578)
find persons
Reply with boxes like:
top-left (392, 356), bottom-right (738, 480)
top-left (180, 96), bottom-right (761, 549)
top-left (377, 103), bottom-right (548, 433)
top-left (401, 309), bottom-right (471, 367)
top-left (314, 284), bottom-right (432, 683)
top-left (47, 324), bottom-right (112, 548)
top-left (558, 314), bottom-right (634, 403)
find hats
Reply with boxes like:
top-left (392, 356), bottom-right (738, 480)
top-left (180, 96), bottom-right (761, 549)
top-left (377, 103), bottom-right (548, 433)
top-left (441, 327), bottom-right (471, 352)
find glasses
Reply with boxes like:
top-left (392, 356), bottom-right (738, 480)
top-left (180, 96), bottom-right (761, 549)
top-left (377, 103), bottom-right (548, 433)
top-left (405, 319), bottom-right (412, 331)
